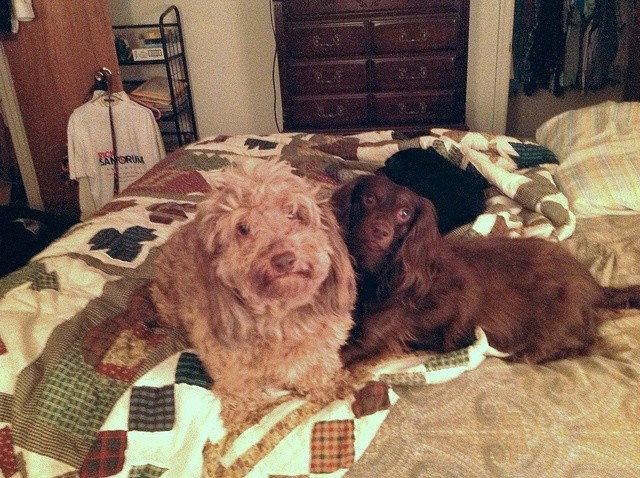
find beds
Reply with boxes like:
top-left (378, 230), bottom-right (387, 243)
top-left (1, 100), bottom-right (640, 478)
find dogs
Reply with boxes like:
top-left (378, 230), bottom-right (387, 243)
top-left (150, 155), bottom-right (357, 429)
top-left (329, 175), bottom-right (640, 366)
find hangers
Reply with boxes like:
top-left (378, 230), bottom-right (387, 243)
top-left (84, 66), bottom-right (164, 120)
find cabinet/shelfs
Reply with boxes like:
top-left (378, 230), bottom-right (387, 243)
top-left (110, 4), bottom-right (199, 152)
top-left (274, 2), bottom-right (476, 136)
